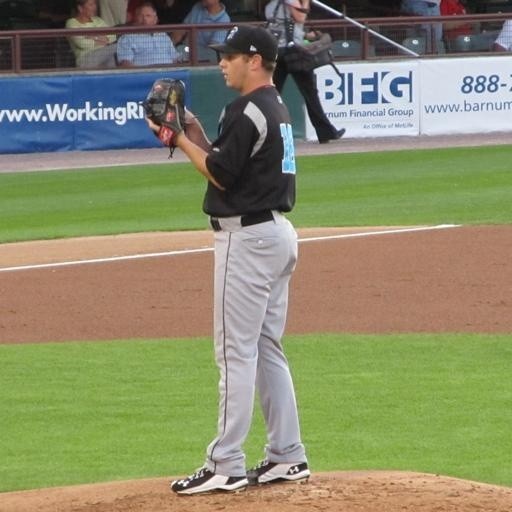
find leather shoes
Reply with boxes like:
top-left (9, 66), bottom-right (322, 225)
top-left (316, 128), bottom-right (346, 144)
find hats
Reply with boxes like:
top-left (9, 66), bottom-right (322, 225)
top-left (209, 25), bottom-right (276, 63)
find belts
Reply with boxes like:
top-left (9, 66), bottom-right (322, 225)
top-left (210, 209), bottom-right (274, 232)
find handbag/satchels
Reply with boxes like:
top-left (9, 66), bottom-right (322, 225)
top-left (277, 33), bottom-right (335, 73)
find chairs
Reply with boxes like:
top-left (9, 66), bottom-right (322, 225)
top-left (457, 33), bottom-right (494, 53)
top-left (403, 37), bottom-right (446, 55)
top-left (176, 44), bottom-right (217, 62)
top-left (331, 40), bottom-right (376, 57)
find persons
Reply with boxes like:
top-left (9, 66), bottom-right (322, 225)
top-left (264, 0), bottom-right (346, 144)
top-left (142, 21), bottom-right (310, 496)
top-left (64, 0), bottom-right (117, 69)
top-left (152, 0), bottom-right (196, 25)
top-left (170, 0), bottom-right (232, 64)
top-left (373, 0), bottom-right (512, 56)
top-left (96, 0), bottom-right (131, 38)
top-left (115, 4), bottom-right (189, 68)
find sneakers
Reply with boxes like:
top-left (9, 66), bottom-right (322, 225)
top-left (170, 458), bottom-right (311, 496)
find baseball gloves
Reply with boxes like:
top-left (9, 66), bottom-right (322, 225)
top-left (142, 77), bottom-right (200, 159)
